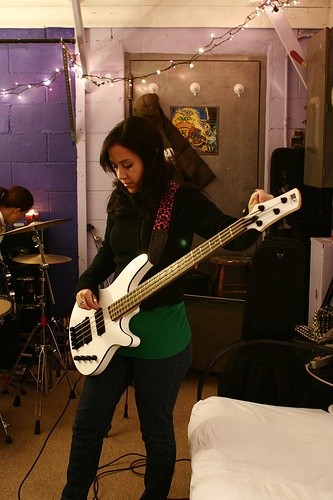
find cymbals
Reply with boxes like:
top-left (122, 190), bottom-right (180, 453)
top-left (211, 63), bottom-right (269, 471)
top-left (12, 277), bottom-right (45, 308)
top-left (13, 253), bottom-right (73, 265)
top-left (0, 219), bottom-right (72, 236)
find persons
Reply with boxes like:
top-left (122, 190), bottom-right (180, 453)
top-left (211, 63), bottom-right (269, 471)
top-left (60, 117), bottom-right (276, 500)
top-left (0, 185), bottom-right (34, 373)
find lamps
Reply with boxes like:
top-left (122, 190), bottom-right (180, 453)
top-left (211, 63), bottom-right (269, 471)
top-left (189, 83), bottom-right (201, 97)
top-left (234, 83), bottom-right (245, 97)
top-left (24, 210), bottom-right (39, 224)
top-left (149, 82), bottom-right (159, 93)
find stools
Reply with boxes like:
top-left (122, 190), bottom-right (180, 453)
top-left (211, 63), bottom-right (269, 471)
top-left (210, 257), bottom-right (250, 303)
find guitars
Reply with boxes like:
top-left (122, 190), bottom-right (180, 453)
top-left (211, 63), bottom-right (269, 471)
top-left (69, 188), bottom-right (302, 376)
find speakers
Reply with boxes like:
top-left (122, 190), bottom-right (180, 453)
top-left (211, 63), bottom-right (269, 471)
top-left (184, 294), bottom-right (246, 375)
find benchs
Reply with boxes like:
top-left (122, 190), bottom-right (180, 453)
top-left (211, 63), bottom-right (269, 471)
top-left (188, 338), bottom-right (333, 499)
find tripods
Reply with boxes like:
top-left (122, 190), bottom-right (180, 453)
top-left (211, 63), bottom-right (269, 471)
top-left (0, 225), bottom-right (78, 435)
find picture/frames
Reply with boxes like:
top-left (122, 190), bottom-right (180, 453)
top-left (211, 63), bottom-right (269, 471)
top-left (169, 105), bottom-right (219, 155)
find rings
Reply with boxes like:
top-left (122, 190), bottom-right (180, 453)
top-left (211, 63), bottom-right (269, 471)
top-left (254, 187), bottom-right (262, 192)
top-left (93, 298), bottom-right (97, 302)
top-left (80, 299), bottom-right (85, 305)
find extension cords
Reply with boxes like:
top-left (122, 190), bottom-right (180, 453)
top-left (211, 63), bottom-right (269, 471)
top-left (309, 355), bottom-right (333, 369)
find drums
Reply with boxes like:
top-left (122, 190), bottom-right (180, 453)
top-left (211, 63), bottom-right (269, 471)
top-left (0, 294), bottom-right (17, 327)
